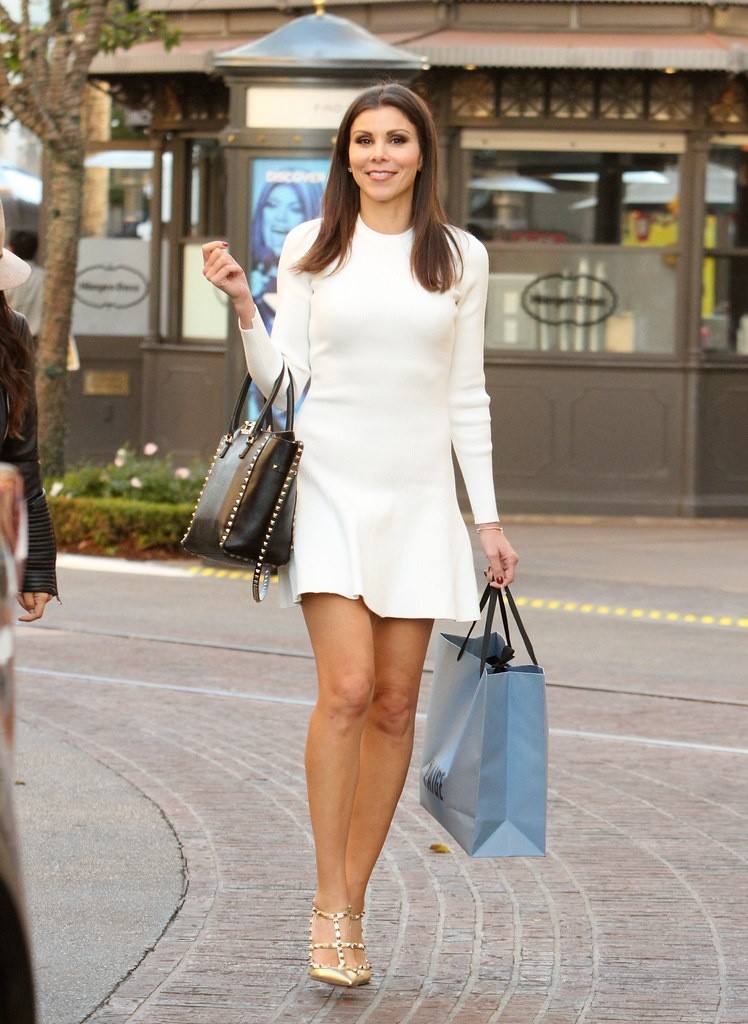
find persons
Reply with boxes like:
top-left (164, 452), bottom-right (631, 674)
top-left (201, 83), bottom-right (520, 987)
top-left (1, 199), bottom-right (61, 745)
top-left (249, 168), bottom-right (320, 336)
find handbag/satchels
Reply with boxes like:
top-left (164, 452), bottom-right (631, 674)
top-left (419, 574), bottom-right (548, 858)
top-left (180, 353), bottom-right (304, 604)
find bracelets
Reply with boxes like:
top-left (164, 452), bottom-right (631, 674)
top-left (475, 527), bottom-right (504, 536)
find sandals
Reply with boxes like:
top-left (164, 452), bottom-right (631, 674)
top-left (346, 912), bottom-right (371, 987)
top-left (308, 900), bottom-right (359, 989)
